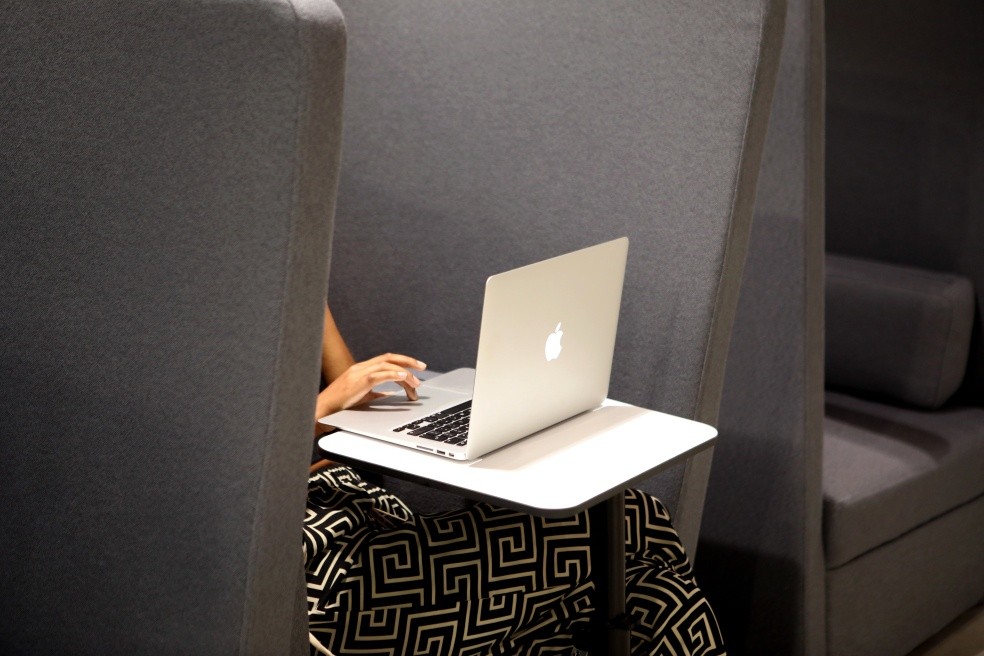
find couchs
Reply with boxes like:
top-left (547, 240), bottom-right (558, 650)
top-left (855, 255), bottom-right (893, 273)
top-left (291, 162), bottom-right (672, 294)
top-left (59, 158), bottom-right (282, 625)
top-left (0, 0), bottom-right (983, 656)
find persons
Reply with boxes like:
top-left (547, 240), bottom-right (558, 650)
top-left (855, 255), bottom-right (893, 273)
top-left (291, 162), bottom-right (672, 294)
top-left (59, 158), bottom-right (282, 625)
top-left (301, 298), bottom-right (727, 656)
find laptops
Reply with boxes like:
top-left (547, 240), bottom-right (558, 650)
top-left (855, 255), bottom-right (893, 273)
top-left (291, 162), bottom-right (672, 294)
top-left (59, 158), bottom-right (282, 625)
top-left (315, 236), bottom-right (630, 461)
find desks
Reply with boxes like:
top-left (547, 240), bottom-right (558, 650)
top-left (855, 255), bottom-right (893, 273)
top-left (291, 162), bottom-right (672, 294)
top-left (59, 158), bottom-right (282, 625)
top-left (315, 399), bottom-right (721, 656)
